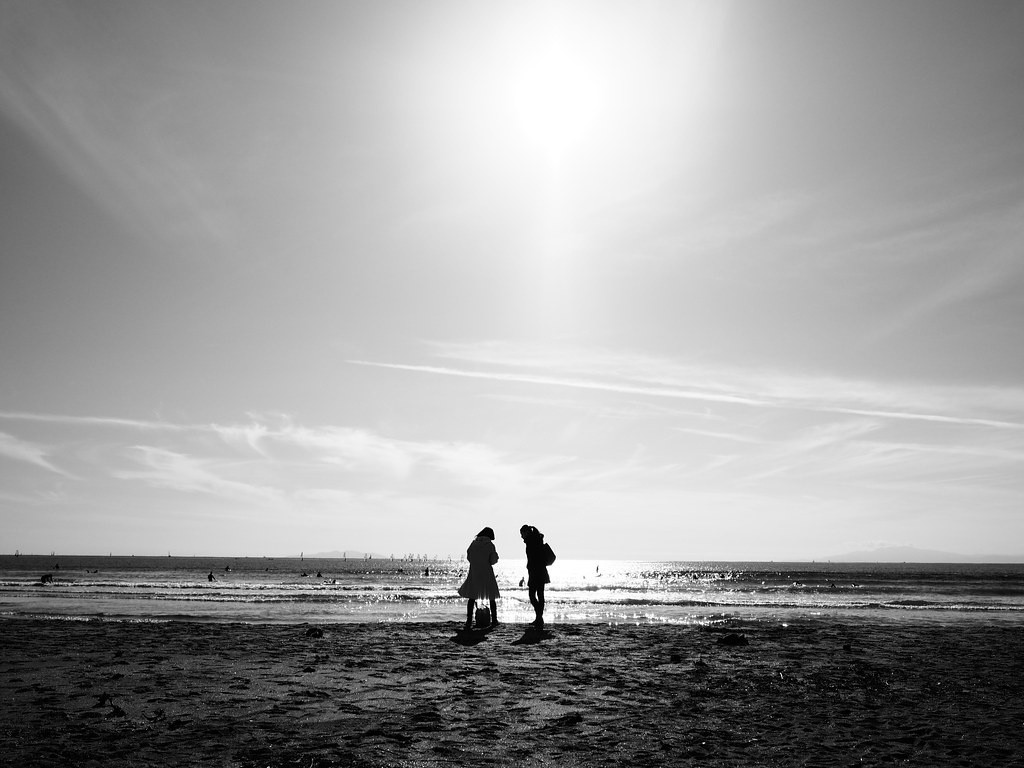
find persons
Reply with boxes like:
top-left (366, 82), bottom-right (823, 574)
top-left (519, 577), bottom-right (525, 587)
top-left (41, 573), bottom-right (53, 583)
top-left (520, 525), bottom-right (551, 626)
top-left (207, 571), bottom-right (216, 582)
top-left (458, 527), bottom-right (501, 627)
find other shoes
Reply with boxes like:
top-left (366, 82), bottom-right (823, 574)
top-left (463, 626), bottom-right (470, 631)
top-left (529, 618), bottom-right (544, 626)
top-left (491, 622), bottom-right (500, 626)
top-left (475, 602), bottom-right (490, 628)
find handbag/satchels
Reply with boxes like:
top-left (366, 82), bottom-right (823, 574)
top-left (541, 543), bottom-right (556, 565)
